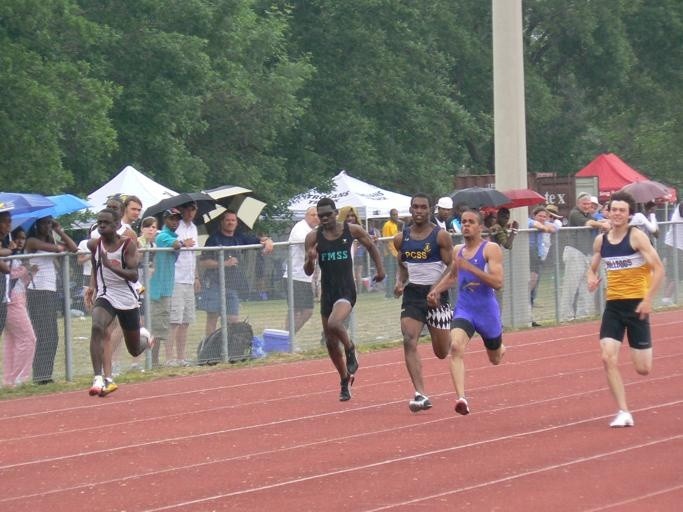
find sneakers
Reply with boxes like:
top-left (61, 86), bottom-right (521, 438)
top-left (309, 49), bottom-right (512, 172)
top-left (609, 410), bottom-right (633, 428)
top-left (89, 327), bottom-right (193, 397)
top-left (340, 339), bottom-right (470, 415)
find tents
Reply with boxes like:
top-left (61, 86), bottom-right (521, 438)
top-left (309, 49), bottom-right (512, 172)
top-left (260, 170), bottom-right (413, 282)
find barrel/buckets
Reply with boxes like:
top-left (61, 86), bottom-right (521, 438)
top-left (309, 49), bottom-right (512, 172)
top-left (262, 328), bottom-right (290, 356)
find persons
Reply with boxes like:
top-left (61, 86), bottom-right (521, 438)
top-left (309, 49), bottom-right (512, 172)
top-left (337, 205), bottom-right (401, 284)
top-left (529, 191), bottom-right (612, 323)
top-left (431, 196), bottom-right (519, 249)
top-left (630, 199), bottom-right (683, 305)
top-left (391, 191), bottom-right (456, 415)
top-left (425, 207), bottom-right (507, 418)
top-left (585, 192), bottom-right (666, 428)
top-left (0, 194), bottom-right (273, 388)
top-left (302, 196), bottom-right (387, 403)
top-left (281, 206), bottom-right (316, 332)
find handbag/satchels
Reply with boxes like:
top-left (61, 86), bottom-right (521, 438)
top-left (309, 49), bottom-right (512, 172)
top-left (238, 272), bottom-right (250, 301)
top-left (197, 322), bottom-right (254, 366)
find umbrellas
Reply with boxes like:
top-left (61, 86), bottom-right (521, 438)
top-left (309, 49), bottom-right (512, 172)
top-left (219, 194), bottom-right (268, 230)
top-left (614, 179), bottom-right (669, 204)
top-left (202, 185), bottom-right (253, 225)
top-left (10, 193), bottom-right (94, 248)
top-left (4, 216), bottom-right (37, 289)
top-left (484, 187), bottom-right (548, 212)
top-left (0, 191), bottom-right (51, 215)
top-left (139, 192), bottom-right (216, 229)
top-left (446, 187), bottom-right (511, 211)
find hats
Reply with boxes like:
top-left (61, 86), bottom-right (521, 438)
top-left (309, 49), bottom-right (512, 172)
top-left (435, 196), bottom-right (453, 209)
top-left (590, 197), bottom-right (604, 212)
top-left (162, 207), bottom-right (185, 219)
top-left (544, 204), bottom-right (564, 219)
